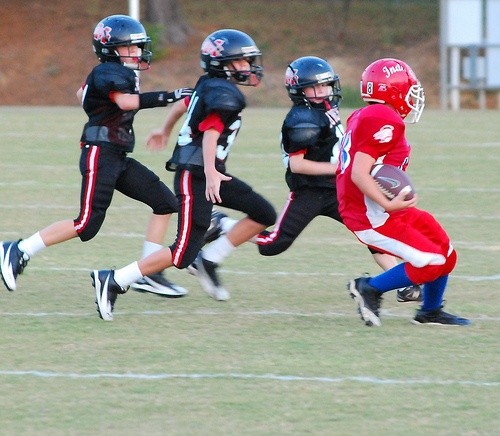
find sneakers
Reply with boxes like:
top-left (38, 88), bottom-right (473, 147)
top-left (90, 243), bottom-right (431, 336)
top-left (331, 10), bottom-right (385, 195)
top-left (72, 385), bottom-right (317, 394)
top-left (186, 209), bottom-right (228, 276)
top-left (90, 265), bottom-right (131, 321)
top-left (410, 299), bottom-right (474, 327)
top-left (346, 271), bottom-right (385, 328)
top-left (129, 270), bottom-right (189, 298)
top-left (0, 238), bottom-right (30, 292)
top-left (190, 242), bottom-right (231, 301)
top-left (396, 284), bottom-right (447, 308)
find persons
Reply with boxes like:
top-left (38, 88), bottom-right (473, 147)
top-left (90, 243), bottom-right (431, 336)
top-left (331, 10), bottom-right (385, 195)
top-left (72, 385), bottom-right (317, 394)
top-left (1, 14), bottom-right (193, 299)
top-left (204, 57), bottom-right (427, 304)
top-left (91, 28), bottom-right (278, 320)
top-left (336, 58), bottom-right (472, 326)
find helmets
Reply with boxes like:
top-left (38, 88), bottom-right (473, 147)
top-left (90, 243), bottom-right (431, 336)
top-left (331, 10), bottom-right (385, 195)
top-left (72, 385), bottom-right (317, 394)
top-left (360, 57), bottom-right (425, 124)
top-left (283, 55), bottom-right (344, 112)
top-left (91, 14), bottom-right (153, 71)
top-left (200, 29), bottom-right (265, 87)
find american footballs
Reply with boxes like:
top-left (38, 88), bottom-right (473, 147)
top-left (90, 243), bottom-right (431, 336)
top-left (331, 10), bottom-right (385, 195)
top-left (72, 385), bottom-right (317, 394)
top-left (370, 164), bottom-right (415, 201)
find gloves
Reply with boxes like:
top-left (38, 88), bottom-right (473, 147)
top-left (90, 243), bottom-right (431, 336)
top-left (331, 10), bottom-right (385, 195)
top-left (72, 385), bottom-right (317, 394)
top-left (166, 87), bottom-right (194, 104)
